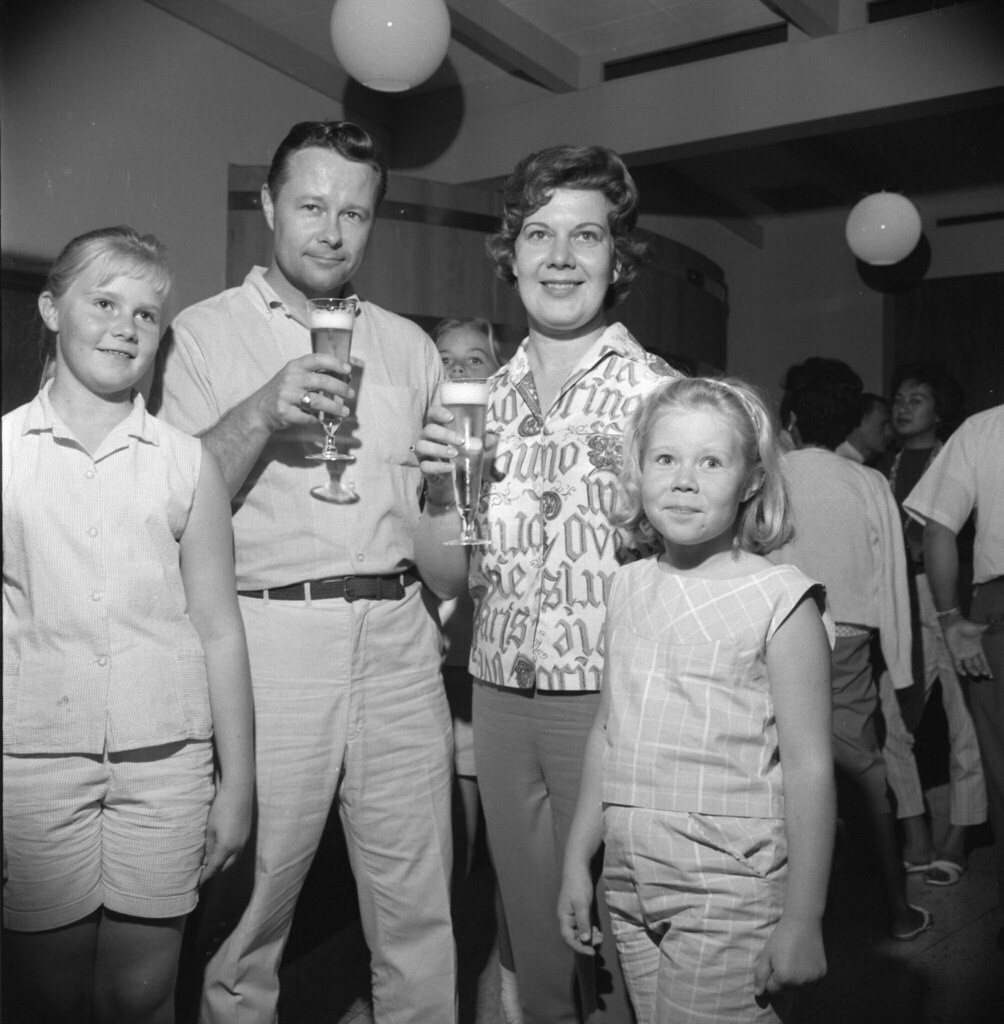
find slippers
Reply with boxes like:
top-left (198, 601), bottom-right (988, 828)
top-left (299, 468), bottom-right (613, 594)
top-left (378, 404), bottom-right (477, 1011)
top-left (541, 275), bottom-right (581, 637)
top-left (925, 860), bottom-right (966, 885)
top-left (889, 904), bottom-right (935, 941)
top-left (902, 859), bottom-right (938, 873)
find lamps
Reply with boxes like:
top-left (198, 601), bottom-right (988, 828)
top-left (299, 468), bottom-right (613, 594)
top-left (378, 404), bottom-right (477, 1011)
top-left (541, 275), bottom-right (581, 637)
top-left (845, 130), bottom-right (921, 264)
top-left (333, 1), bottom-right (453, 93)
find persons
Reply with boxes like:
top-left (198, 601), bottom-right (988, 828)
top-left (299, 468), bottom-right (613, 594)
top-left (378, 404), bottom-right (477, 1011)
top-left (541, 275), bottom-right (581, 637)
top-left (556, 378), bottom-right (836, 1024)
top-left (437, 315), bottom-right (524, 1023)
top-left (771, 356), bottom-right (1003, 948)
top-left (2, 226), bottom-right (257, 1024)
top-left (415, 143), bottom-right (684, 1024)
top-left (148, 120), bottom-right (447, 1024)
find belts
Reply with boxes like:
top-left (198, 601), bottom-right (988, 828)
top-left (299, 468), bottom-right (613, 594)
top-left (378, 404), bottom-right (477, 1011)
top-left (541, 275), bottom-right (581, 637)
top-left (237, 568), bottom-right (419, 603)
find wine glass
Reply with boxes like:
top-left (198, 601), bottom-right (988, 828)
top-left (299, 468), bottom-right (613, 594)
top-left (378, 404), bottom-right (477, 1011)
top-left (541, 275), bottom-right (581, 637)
top-left (304, 299), bottom-right (359, 463)
top-left (442, 379), bottom-right (493, 547)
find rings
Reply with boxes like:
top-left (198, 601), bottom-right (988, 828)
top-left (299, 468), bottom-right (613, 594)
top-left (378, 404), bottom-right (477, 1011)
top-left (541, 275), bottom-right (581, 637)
top-left (300, 392), bottom-right (311, 411)
top-left (411, 443), bottom-right (422, 458)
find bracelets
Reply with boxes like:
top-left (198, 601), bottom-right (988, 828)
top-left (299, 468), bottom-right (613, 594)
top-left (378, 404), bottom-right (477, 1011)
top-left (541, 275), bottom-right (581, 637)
top-left (425, 489), bottom-right (455, 508)
top-left (937, 605), bottom-right (961, 617)
